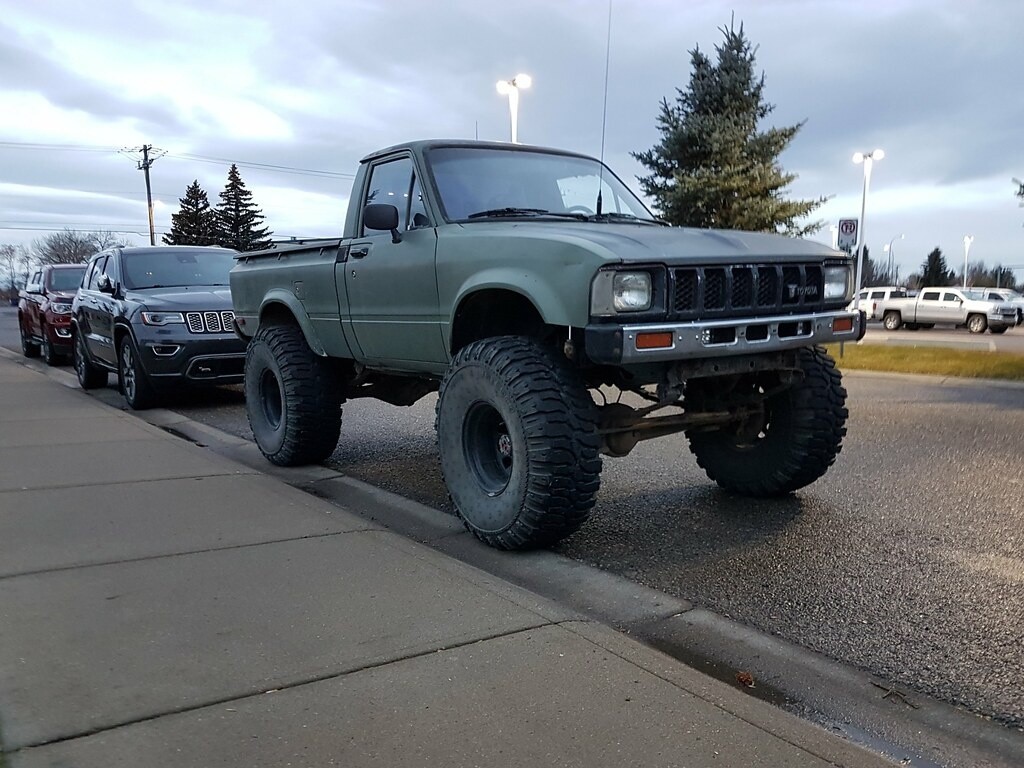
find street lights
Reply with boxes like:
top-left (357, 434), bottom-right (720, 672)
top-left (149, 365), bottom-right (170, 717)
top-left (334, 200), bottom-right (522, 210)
top-left (494, 72), bottom-right (532, 148)
top-left (852, 148), bottom-right (886, 310)
top-left (963, 234), bottom-right (975, 287)
top-left (882, 235), bottom-right (905, 287)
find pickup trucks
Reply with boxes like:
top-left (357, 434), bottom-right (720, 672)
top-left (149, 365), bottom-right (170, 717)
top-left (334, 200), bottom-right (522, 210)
top-left (848, 287), bottom-right (1024, 335)
top-left (230, 139), bottom-right (867, 554)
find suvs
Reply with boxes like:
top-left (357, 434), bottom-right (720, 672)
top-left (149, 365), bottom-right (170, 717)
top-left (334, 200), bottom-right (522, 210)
top-left (70, 242), bottom-right (248, 411)
top-left (18, 262), bottom-right (93, 367)
